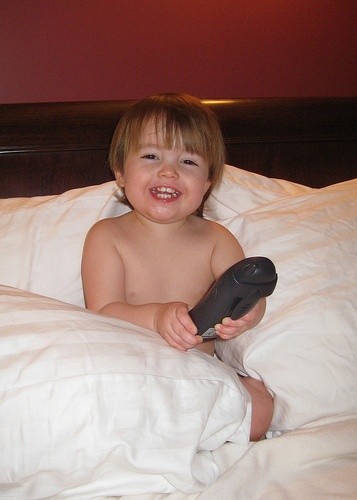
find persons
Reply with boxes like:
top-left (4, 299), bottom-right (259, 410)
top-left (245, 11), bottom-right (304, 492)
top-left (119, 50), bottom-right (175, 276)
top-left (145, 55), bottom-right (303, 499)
top-left (79, 91), bottom-right (276, 445)
